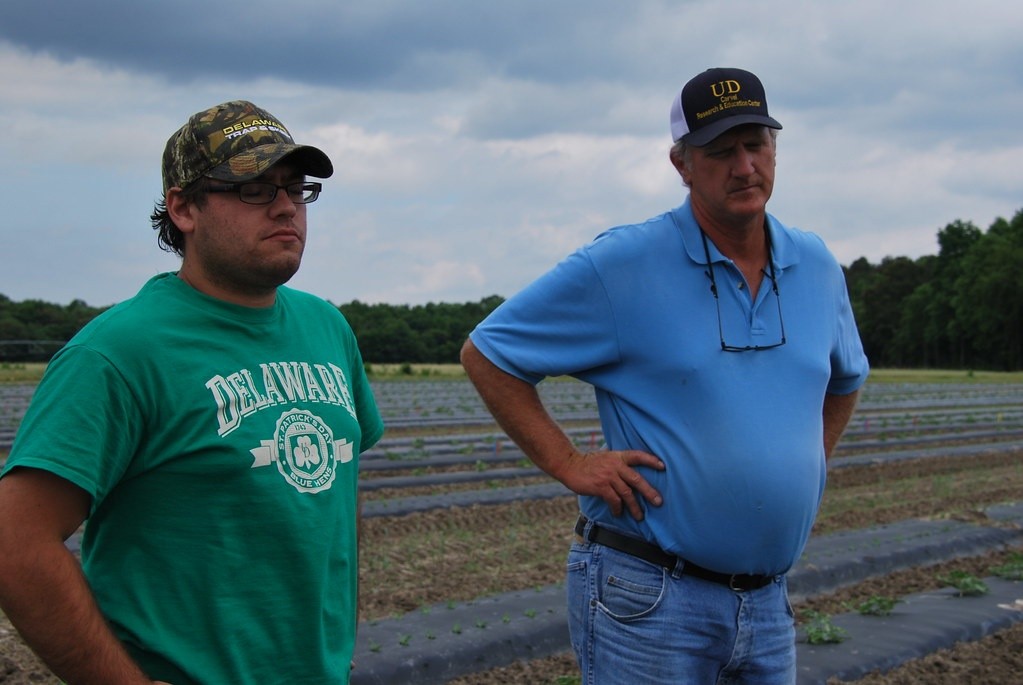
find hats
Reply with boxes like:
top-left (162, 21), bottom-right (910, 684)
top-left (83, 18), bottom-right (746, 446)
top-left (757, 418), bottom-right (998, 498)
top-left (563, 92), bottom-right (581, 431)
top-left (161, 100), bottom-right (333, 200)
top-left (670, 68), bottom-right (783, 147)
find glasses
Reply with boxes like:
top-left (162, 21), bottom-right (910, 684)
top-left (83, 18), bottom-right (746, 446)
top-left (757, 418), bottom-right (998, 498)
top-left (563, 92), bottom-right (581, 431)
top-left (193, 181), bottom-right (322, 205)
top-left (705, 268), bottom-right (786, 352)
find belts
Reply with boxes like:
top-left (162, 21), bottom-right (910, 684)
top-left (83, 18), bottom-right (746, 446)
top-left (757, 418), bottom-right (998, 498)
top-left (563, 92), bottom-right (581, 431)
top-left (574, 515), bottom-right (772, 591)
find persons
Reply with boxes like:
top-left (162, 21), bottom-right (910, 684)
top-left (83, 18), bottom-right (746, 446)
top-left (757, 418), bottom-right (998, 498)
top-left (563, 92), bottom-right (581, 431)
top-left (460, 67), bottom-right (870, 683)
top-left (2, 99), bottom-right (389, 683)
